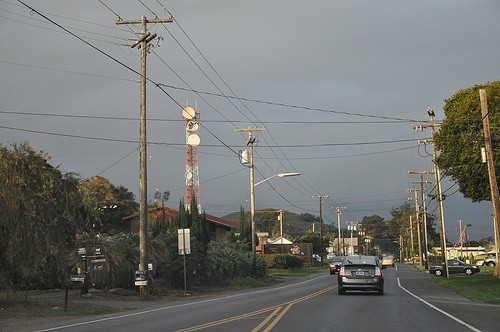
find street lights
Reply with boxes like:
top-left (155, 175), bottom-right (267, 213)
top-left (246, 172), bottom-right (301, 277)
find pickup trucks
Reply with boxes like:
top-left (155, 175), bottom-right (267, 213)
top-left (429, 259), bottom-right (481, 276)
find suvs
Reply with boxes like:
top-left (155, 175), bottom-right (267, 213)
top-left (337, 255), bottom-right (384, 296)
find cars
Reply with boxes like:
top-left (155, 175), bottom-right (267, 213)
top-left (382, 255), bottom-right (396, 267)
top-left (329, 257), bottom-right (343, 275)
top-left (485, 254), bottom-right (500, 266)
top-left (404, 257), bottom-right (426, 266)
top-left (327, 252), bottom-right (335, 260)
